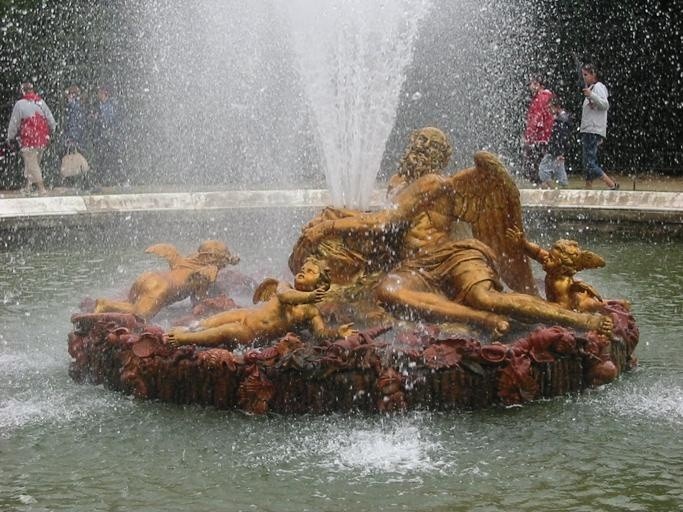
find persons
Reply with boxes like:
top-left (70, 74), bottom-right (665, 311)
top-left (299, 124), bottom-right (614, 344)
top-left (7, 80), bottom-right (58, 196)
top-left (515, 74), bottom-right (558, 189)
top-left (502, 224), bottom-right (631, 316)
top-left (575, 63), bottom-right (623, 190)
top-left (50, 82), bottom-right (102, 194)
top-left (537, 95), bottom-right (572, 188)
top-left (90, 84), bottom-right (133, 188)
top-left (165, 254), bottom-right (360, 350)
top-left (95, 239), bottom-right (244, 324)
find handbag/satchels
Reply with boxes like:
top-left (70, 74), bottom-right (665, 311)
top-left (59, 144), bottom-right (90, 177)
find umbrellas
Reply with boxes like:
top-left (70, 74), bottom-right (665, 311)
top-left (567, 47), bottom-right (596, 112)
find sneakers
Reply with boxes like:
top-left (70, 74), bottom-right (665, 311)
top-left (610, 182), bottom-right (620, 191)
top-left (19, 188), bottom-right (48, 197)
